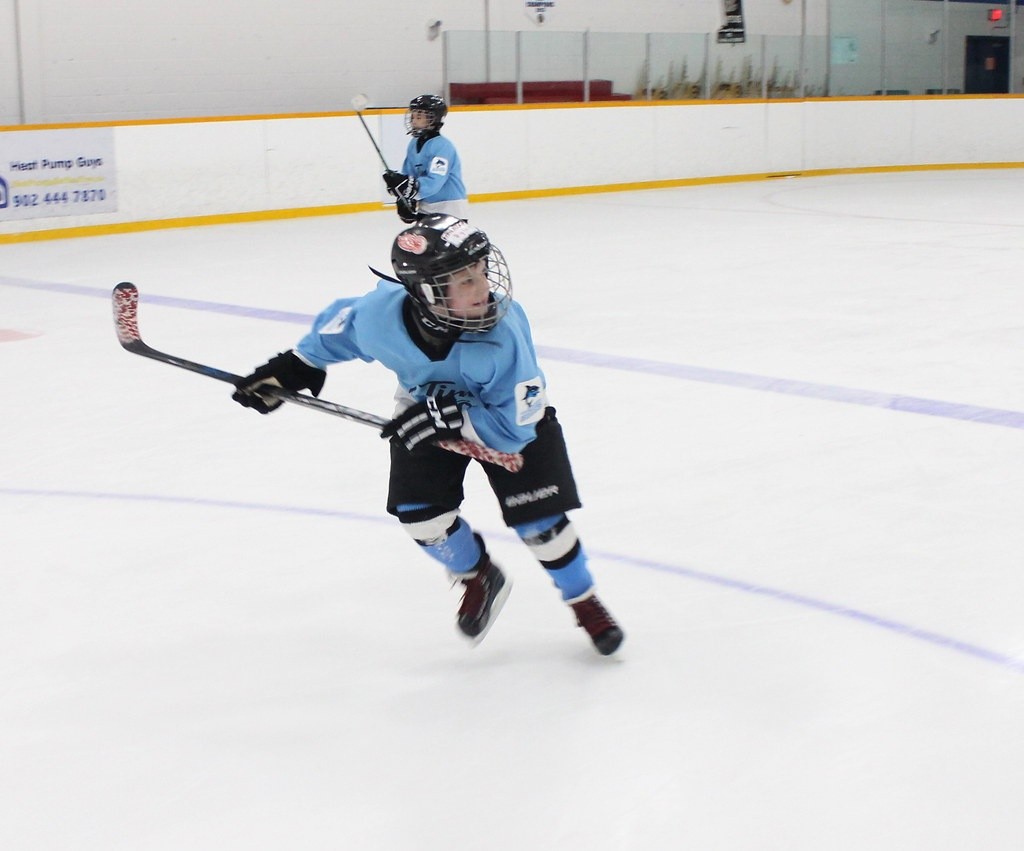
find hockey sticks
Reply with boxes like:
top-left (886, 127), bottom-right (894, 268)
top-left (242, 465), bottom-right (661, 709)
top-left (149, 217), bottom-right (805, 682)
top-left (352, 94), bottom-right (390, 171)
top-left (112, 283), bottom-right (524, 474)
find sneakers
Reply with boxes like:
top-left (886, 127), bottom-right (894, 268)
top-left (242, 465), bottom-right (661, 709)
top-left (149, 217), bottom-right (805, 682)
top-left (450, 532), bottom-right (513, 644)
top-left (574, 594), bottom-right (623, 656)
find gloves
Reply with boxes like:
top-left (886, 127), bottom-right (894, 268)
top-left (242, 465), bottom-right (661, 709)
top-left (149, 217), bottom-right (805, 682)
top-left (380, 392), bottom-right (465, 454)
top-left (396, 197), bottom-right (419, 224)
top-left (383, 169), bottom-right (419, 200)
top-left (230, 349), bottom-right (326, 414)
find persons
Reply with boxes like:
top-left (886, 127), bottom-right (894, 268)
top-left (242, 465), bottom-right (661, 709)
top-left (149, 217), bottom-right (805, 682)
top-left (383, 96), bottom-right (467, 235)
top-left (232, 213), bottom-right (627, 657)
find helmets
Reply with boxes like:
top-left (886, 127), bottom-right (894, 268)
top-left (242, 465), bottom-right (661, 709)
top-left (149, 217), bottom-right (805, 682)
top-left (390, 210), bottom-right (511, 330)
top-left (405, 94), bottom-right (448, 138)
